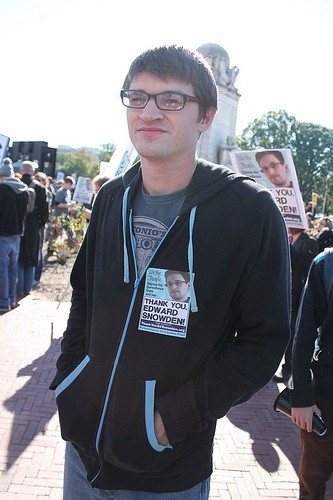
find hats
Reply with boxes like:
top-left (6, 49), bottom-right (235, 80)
top-left (20, 161), bottom-right (36, 171)
top-left (0, 157), bottom-right (15, 178)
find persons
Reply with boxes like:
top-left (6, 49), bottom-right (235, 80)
top-left (254, 150), bottom-right (293, 188)
top-left (273, 202), bottom-right (333, 500)
top-left (48, 45), bottom-right (292, 500)
top-left (0, 153), bottom-right (110, 312)
top-left (164, 270), bottom-right (191, 304)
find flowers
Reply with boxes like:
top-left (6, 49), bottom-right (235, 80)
top-left (47, 201), bottom-right (87, 267)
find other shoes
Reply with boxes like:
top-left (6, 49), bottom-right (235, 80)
top-left (0, 303), bottom-right (17, 312)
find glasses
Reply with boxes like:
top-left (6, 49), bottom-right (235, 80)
top-left (119, 89), bottom-right (201, 111)
top-left (166, 280), bottom-right (185, 287)
top-left (261, 162), bottom-right (281, 173)
